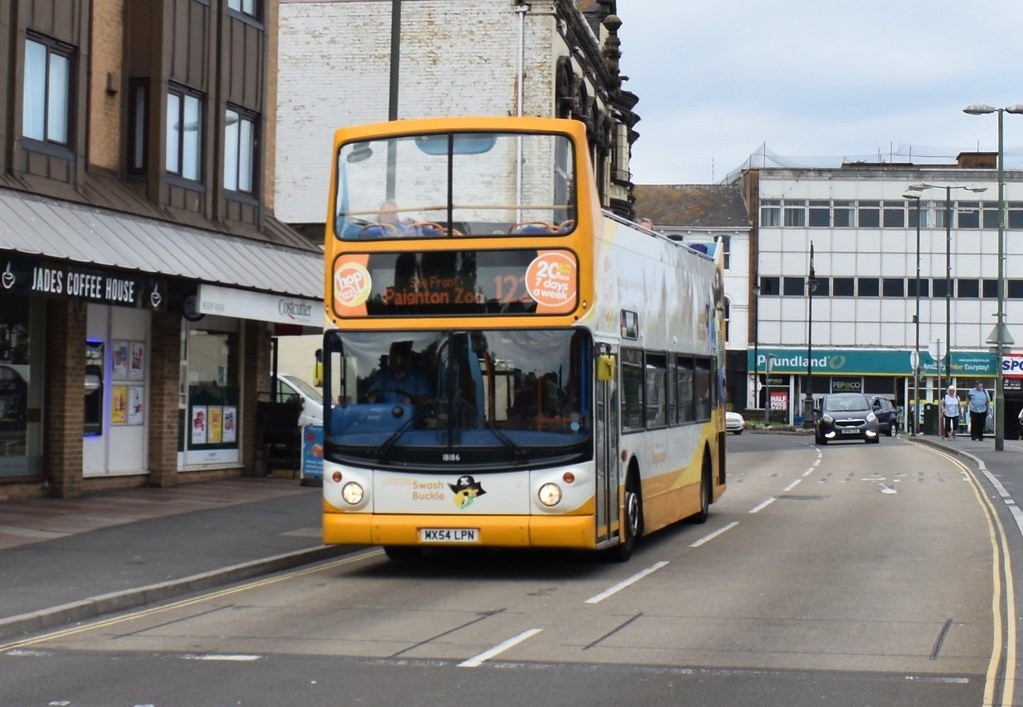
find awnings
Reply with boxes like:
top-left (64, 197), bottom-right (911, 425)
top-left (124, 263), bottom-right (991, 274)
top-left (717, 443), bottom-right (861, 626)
top-left (0, 196), bottom-right (325, 328)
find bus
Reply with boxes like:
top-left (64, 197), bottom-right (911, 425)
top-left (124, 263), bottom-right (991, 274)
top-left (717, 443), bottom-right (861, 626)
top-left (312, 117), bottom-right (735, 559)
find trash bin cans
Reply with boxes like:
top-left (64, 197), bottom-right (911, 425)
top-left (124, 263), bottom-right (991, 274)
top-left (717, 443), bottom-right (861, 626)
top-left (924, 403), bottom-right (938, 435)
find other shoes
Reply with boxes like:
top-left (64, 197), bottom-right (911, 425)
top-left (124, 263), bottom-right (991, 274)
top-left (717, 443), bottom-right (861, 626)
top-left (944, 437), bottom-right (948, 440)
top-left (952, 432), bottom-right (957, 439)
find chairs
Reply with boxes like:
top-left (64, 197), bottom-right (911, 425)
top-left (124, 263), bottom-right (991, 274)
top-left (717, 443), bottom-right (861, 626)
top-left (403, 221), bottom-right (444, 237)
top-left (510, 220), bottom-right (553, 235)
top-left (557, 220), bottom-right (576, 235)
top-left (357, 223), bottom-right (400, 237)
top-left (443, 227), bottom-right (464, 237)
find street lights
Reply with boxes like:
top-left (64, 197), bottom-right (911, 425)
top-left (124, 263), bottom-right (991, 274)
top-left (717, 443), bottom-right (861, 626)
top-left (907, 182), bottom-right (988, 436)
top-left (961, 103), bottom-right (1022, 449)
top-left (765, 353), bottom-right (778, 428)
top-left (903, 192), bottom-right (922, 437)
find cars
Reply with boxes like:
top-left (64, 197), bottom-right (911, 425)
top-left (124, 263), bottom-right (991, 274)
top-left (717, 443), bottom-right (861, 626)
top-left (866, 394), bottom-right (898, 437)
top-left (723, 413), bottom-right (746, 435)
top-left (812, 393), bottom-right (882, 445)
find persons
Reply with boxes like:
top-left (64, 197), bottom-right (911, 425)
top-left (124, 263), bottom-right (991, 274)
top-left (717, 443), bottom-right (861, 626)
top-left (378, 201), bottom-right (398, 226)
top-left (365, 353), bottom-right (432, 404)
top-left (966, 380), bottom-right (991, 441)
top-left (941, 385), bottom-right (962, 440)
